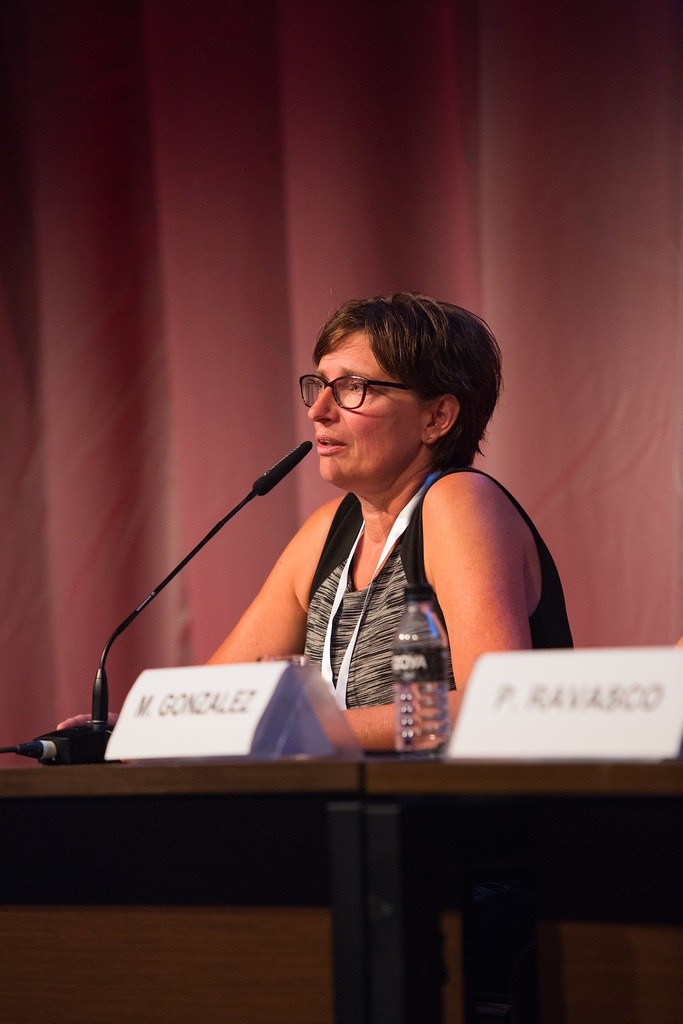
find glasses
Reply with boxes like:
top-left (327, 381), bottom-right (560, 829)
top-left (298, 373), bottom-right (411, 410)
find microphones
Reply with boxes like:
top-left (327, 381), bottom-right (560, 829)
top-left (39, 439), bottom-right (314, 767)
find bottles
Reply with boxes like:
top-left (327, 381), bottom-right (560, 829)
top-left (390, 582), bottom-right (453, 754)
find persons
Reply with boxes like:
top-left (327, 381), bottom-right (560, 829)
top-left (57, 291), bottom-right (572, 758)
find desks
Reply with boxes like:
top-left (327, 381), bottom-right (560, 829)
top-left (0, 761), bottom-right (683, 1024)
